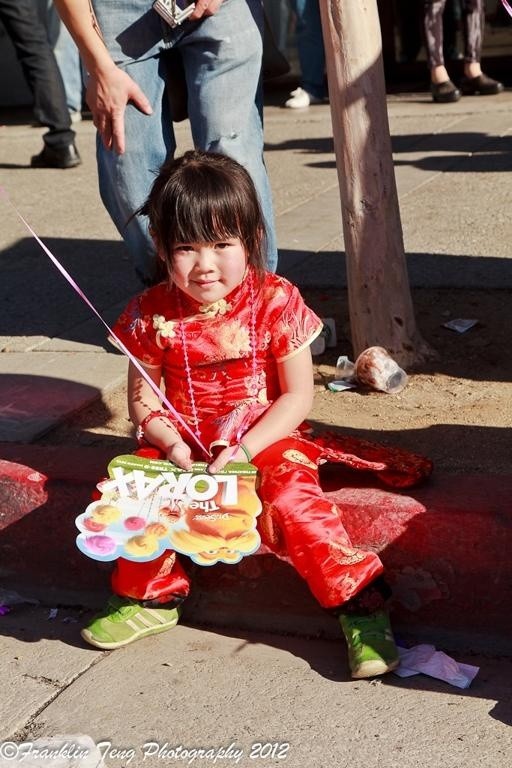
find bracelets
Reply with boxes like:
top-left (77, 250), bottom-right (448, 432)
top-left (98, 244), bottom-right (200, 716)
top-left (132, 408), bottom-right (170, 446)
top-left (235, 441), bottom-right (253, 462)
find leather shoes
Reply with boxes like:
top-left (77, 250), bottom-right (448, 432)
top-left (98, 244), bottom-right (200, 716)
top-left (459, 72), bottom-right (505, 94)
top-left (430, 78), bottom-right (461, 101)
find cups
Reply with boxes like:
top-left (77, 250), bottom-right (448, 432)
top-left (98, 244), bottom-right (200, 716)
top-left (355, 345), bottom-right (408, 394)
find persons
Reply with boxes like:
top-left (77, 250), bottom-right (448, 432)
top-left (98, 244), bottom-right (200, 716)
top-left (0, 2), bottom-right (509, 169)
top-left (78, 150), bottom-right (436, 680)
top-left (54, 0), bottom-right (281, 286)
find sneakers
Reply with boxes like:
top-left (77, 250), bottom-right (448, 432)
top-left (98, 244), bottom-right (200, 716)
top-left (340, 601), bottom-right (399, 679)
top-left (281, 84), bottom-right (330, 108)
top-left (28, 138), bottom-right (81, 168)
top-left (80, 590), bottom-right (180, 651)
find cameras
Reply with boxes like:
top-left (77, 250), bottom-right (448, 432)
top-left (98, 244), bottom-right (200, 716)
top-left (152, 0), bottom-right (197, 28)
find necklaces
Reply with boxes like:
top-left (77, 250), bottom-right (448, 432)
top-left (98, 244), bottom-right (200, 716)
top-left (166, 276), bottom-right (256, 443)
